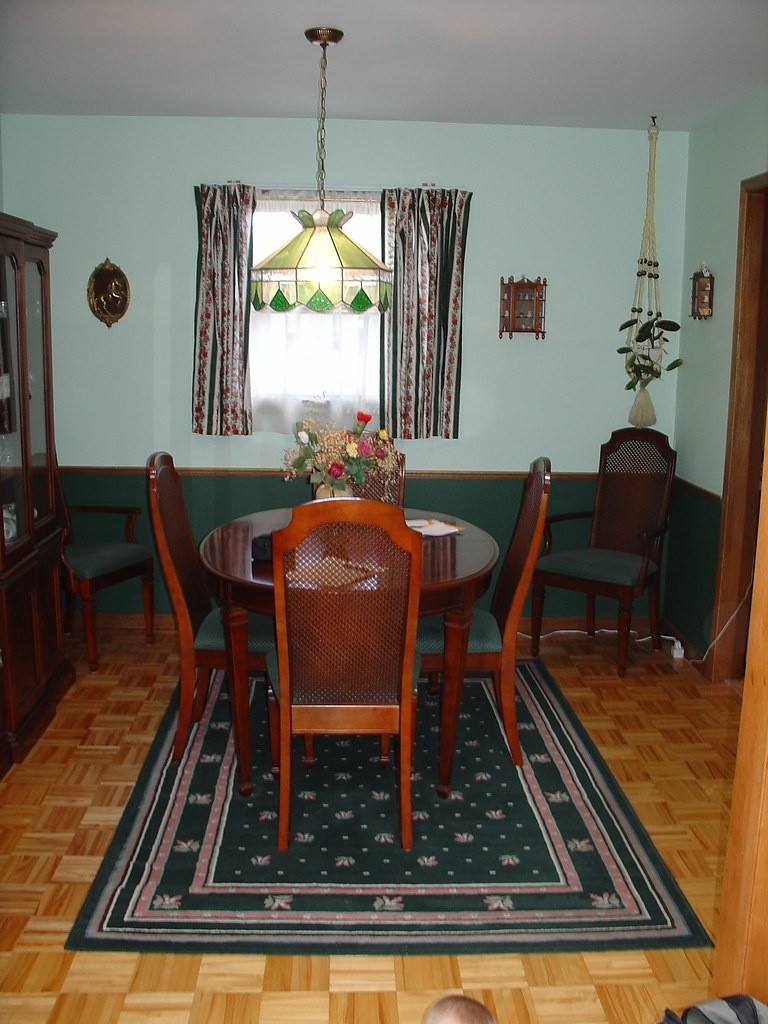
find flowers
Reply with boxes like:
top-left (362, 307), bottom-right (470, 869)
top-left (280, 409), bottom-right (393, 490)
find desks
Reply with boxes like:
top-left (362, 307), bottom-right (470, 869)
top-left (198, 506), bottom-right (501, 800)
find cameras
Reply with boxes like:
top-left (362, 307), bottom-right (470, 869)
top-left (251, 533), bottom-right (273, 561)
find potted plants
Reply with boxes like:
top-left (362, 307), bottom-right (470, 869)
top-left (616, 315), bottom-right (684, 390)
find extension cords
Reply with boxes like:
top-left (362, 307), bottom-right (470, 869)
top-left (670, 645), bottom-right (684, 658)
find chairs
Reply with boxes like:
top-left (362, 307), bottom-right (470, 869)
top-left (149, 452), bottom-right (313, 766)
top-left (265, 496), bottom-right (420, 852)
top-left (381, 456), bottom-right (551, 767)
top-left (59, 476), bottom-right (156, 671)
top-left (312, 450), bottom-right (405, 508)
top-left (531, 429), bottom-right (677, 677)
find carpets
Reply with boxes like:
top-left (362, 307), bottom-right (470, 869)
top-left (62, 659), bottom-right (715, 956)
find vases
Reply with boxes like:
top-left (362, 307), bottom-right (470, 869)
top-left (316, 480), bottom-right (353, 500)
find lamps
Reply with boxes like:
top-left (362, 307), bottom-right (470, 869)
top-left (250, 26), bottom-right (395, 312)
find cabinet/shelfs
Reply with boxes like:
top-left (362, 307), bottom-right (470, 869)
top-left (688, 272), bottom-right (715, 320)
top-left (0, 211), bottom-right (77, 781)
top-left (499, 275), bottom-right (548, 340)
